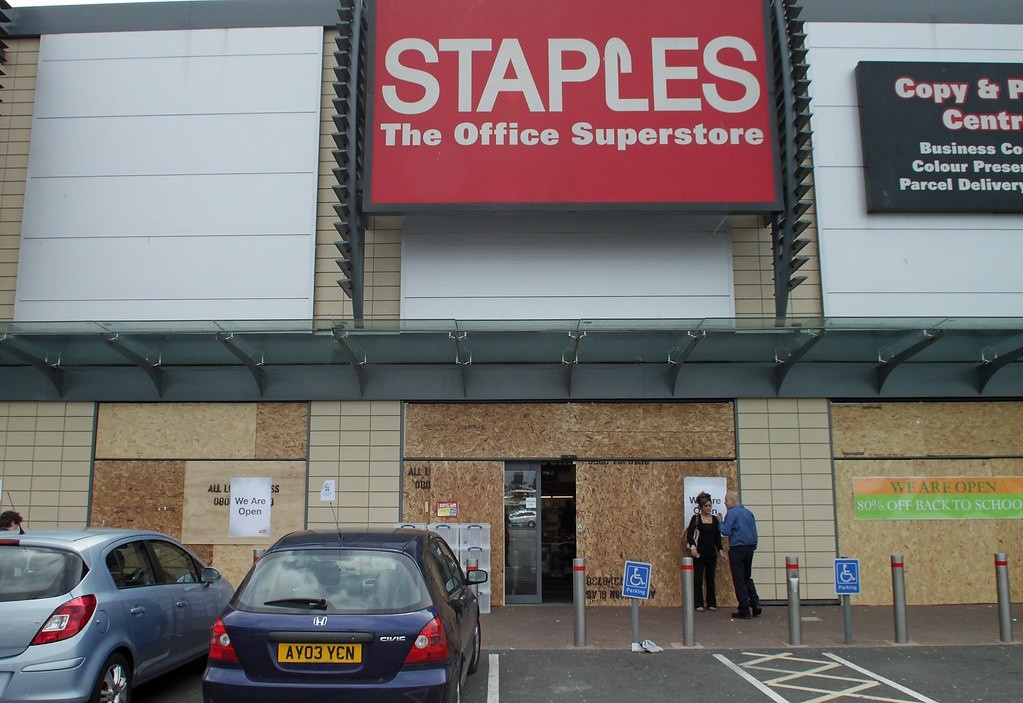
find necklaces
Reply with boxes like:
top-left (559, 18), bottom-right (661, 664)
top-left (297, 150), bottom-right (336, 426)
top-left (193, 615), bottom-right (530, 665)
top-left (706, 519), bottom-right (708, 522)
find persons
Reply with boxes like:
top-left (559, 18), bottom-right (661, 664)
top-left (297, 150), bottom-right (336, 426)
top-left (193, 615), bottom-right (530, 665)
top-left (716, 491), bottom-right (762, 619)
top-left (687, 491), bottom-right (728, 612)
top-left (0, 510), bottom-right (27, 579)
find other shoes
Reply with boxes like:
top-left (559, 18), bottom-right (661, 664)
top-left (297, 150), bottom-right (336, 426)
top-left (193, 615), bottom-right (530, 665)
top-left (708, 605), bottom-right (717, 610)
top-left (753, 606), bottom-right (761, 616)
top-left (732, 612), bottom-right (750, 619)
top-left (695, 607), bottom-right (704, 611)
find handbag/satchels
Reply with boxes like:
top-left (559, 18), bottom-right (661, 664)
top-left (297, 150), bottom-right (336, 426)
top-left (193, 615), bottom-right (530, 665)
top-left (682, 515), bottom-right (700, 550)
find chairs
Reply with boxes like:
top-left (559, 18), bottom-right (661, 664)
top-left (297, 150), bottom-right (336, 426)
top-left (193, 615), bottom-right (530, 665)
top-left (301, 560), bottom-right (351, 606)
top-left (105, 550), bottom-right (130, 588)
top-left (365, 569), bottom-right (415, 606)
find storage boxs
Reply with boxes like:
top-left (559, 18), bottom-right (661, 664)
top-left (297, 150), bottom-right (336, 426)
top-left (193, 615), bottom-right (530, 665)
top-left (395, 522), bottom-right (491, 613)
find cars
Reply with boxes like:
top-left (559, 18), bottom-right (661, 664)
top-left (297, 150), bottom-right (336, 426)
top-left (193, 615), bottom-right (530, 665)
top-left (201, 528), bottom-right (489, 702)
top-left (0, 528), bottom-right (237, 703)
top-left (508, 510), bottom-right (536, 528)
